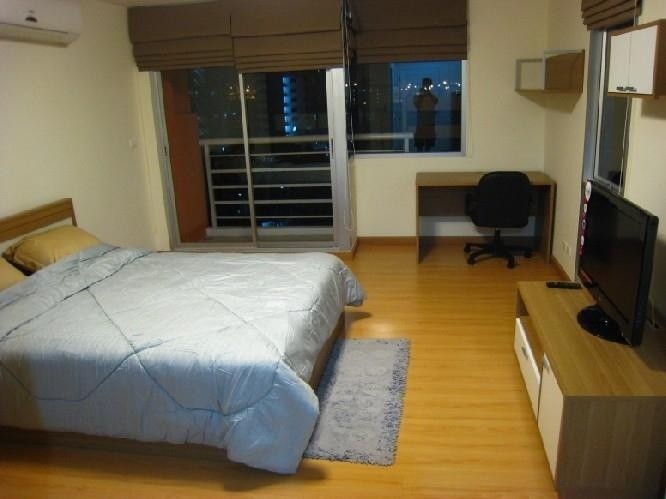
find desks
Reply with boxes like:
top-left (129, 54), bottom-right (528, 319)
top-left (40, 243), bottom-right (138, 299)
top-left (415, 171), bottom-right (559, 264)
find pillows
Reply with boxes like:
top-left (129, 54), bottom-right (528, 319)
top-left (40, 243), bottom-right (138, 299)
top-left (0, 226), bottom-right (105, 292)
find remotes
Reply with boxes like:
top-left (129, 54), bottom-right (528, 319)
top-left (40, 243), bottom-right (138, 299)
top-left (546, 282), bottom-right (582, 289)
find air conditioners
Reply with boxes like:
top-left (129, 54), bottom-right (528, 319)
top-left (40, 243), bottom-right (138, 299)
top-left (1, 1), bottom-right (85, 49)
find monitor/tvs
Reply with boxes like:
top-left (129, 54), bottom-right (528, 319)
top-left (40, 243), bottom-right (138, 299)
top-left (577, 179), bottom-right (659, 349)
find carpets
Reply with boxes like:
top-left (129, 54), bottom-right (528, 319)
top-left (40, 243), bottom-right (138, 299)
top-left (302, 336), bottom-right (412, 467)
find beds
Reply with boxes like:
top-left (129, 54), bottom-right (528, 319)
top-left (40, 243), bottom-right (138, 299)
top-left (0, 196), bottom-right (344, 474)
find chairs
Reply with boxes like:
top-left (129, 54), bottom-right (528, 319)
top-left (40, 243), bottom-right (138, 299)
top-left (465, 173), bottom-right (532, 270)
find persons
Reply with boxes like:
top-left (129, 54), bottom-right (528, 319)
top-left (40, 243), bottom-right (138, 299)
top-left (413, 78), bottom-right (438, 152)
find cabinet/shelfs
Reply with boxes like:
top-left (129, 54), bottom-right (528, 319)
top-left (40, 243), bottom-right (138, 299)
top-left (608, 26), bottom-right (666, 95)
top-left (511, 280), bottom-right (666, 498)
